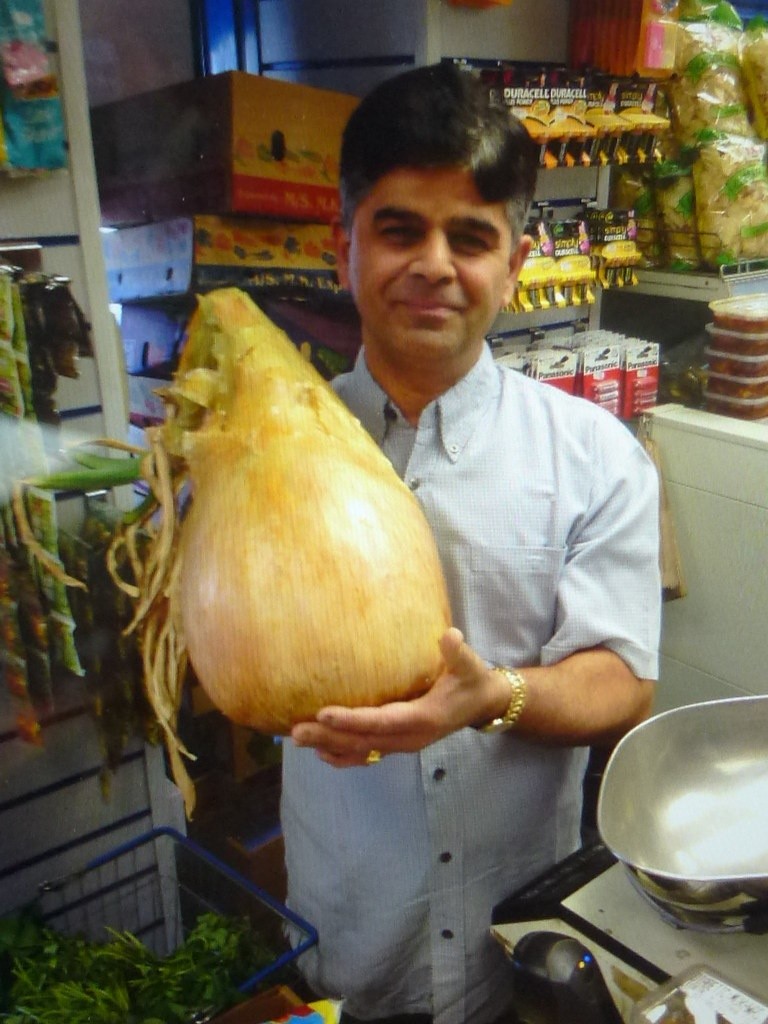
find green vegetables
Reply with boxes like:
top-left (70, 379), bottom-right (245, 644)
top-left (1, 906), bottom-right (280, 1024)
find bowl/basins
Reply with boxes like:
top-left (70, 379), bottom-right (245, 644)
top-left (595, 695), bottom-right (768, 935)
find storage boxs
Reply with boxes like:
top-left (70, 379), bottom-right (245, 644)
top-left (90, 68), bottom-right (367, 431)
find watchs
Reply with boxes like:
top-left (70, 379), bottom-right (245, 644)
top-left (469, 666), bottom-right (531, 736)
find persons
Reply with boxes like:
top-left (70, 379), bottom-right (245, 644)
top-left (280, 63), bottom-right (663, 1024)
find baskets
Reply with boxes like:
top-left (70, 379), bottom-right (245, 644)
top-left (1, 828), bottom-right (319, 1023)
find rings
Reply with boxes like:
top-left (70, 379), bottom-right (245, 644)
top-left (364, 749), bottom-right (382, 765)
